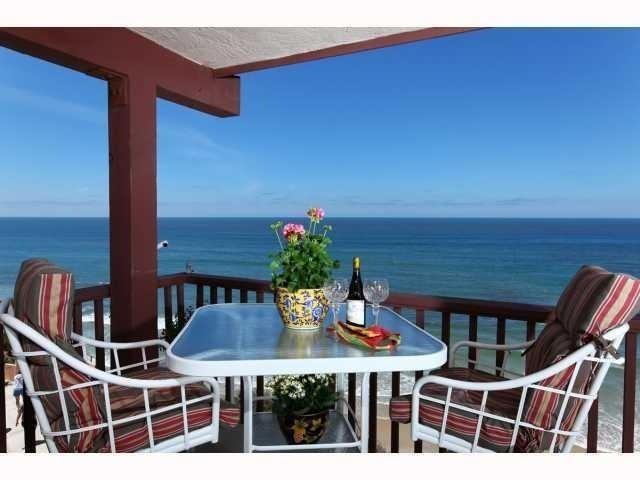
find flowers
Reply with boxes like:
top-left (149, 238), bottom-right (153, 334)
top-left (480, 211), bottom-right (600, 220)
top-left (267, 206), bottom-right (341, 288)
top-left (264, 374), bottom-right (342, 414)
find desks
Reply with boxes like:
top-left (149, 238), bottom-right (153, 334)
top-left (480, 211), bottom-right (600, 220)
top-left (166, 303), bottom-right (448, 453)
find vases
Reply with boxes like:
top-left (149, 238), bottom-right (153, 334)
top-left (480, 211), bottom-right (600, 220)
top-left (275, 286), bottom-right (331, 330)
top-left (276, 408), bottom-right (330, 444)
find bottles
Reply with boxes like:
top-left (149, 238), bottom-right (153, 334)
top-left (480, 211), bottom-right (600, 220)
top-left (346, 257), bottom-right (366, 327)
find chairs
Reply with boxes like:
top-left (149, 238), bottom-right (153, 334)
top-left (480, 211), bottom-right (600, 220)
top-left (389, 263), bottom-right (640, 453)
top-left (1, 257), bottom-right (240, 453)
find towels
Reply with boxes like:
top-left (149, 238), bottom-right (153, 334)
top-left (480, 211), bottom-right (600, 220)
top-left (335, 319), bottom-right (401, 350)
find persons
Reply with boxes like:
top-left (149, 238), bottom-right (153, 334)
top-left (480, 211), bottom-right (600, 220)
top-left (15, 399), bottom-right (37, 451)
top-left (13, 370), bottom-right (25, 408)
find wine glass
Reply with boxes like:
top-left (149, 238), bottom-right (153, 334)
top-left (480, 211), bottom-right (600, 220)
top-left (362, 278), bottom-right (390, 328)
top-left (322, 274), bottom-right (350, 327)
